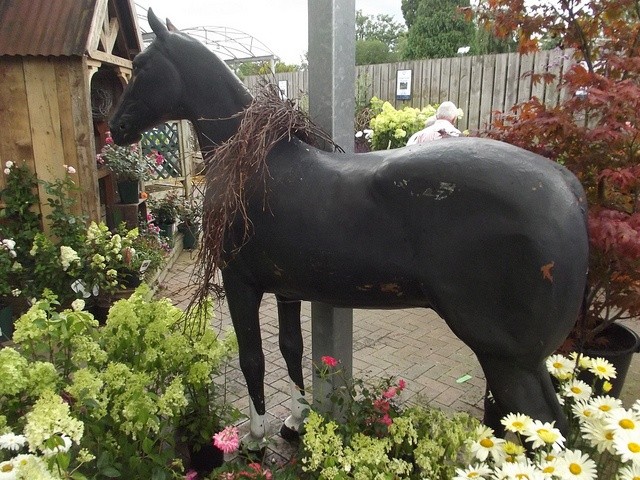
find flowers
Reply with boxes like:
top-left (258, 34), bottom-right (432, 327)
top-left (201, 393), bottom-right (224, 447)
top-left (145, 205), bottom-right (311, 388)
top-left (97, 130), bottom-right (164, 180)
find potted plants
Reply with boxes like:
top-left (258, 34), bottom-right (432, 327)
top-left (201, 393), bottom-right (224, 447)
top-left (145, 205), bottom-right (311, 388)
top-left (438, 0), bottom-right (639, 459)
top-left (175, 198), bottom-right (203, 248)
top-left (147, 189), bottom-right (177, 249)
top-left (146, 298), bottom-right (241, 471)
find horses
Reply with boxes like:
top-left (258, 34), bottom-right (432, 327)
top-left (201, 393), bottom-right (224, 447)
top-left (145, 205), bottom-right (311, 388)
top-left (108, 7), bottom-right (590, 465)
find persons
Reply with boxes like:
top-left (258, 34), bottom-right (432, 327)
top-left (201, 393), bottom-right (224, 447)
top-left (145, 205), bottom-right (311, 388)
top-left (144, 145), bottom-right (163, 171)
top-left (169, 144), bottom-right (176, 154)
top-left (404, 99), bottom-right (462, 146)
top-left (158, 145), bottom-right (168, 155)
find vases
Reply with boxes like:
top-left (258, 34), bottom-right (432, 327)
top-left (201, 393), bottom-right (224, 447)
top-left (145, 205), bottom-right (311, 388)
top-left (117, 179), bottom-right (139, 203)
